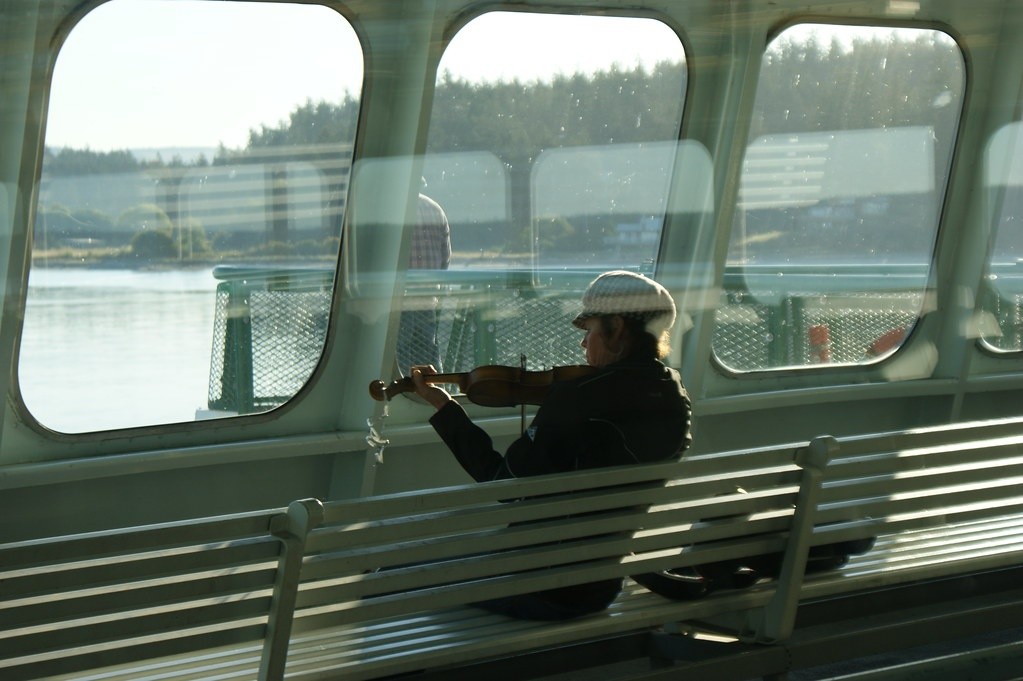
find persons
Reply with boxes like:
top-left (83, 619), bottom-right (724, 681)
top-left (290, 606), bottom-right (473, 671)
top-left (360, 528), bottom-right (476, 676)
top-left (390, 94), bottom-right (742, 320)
top-left (410, 269), bottom-right (695, 622)
top-left (394, 169), bottom-right (452, 397)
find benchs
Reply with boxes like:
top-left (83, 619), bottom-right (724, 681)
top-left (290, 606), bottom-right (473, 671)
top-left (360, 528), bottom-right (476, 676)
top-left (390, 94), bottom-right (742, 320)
top-left (0, 412), bottom-right (1023, 681)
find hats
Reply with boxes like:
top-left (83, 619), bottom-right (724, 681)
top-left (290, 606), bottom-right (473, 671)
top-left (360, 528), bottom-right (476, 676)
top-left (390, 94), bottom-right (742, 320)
top-left (572, 271), bottom-right (677, 340)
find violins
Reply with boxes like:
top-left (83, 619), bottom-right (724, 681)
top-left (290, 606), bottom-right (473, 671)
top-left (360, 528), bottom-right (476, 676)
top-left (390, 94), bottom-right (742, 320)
top-left (369, 364), bottom-right (600, 408)
